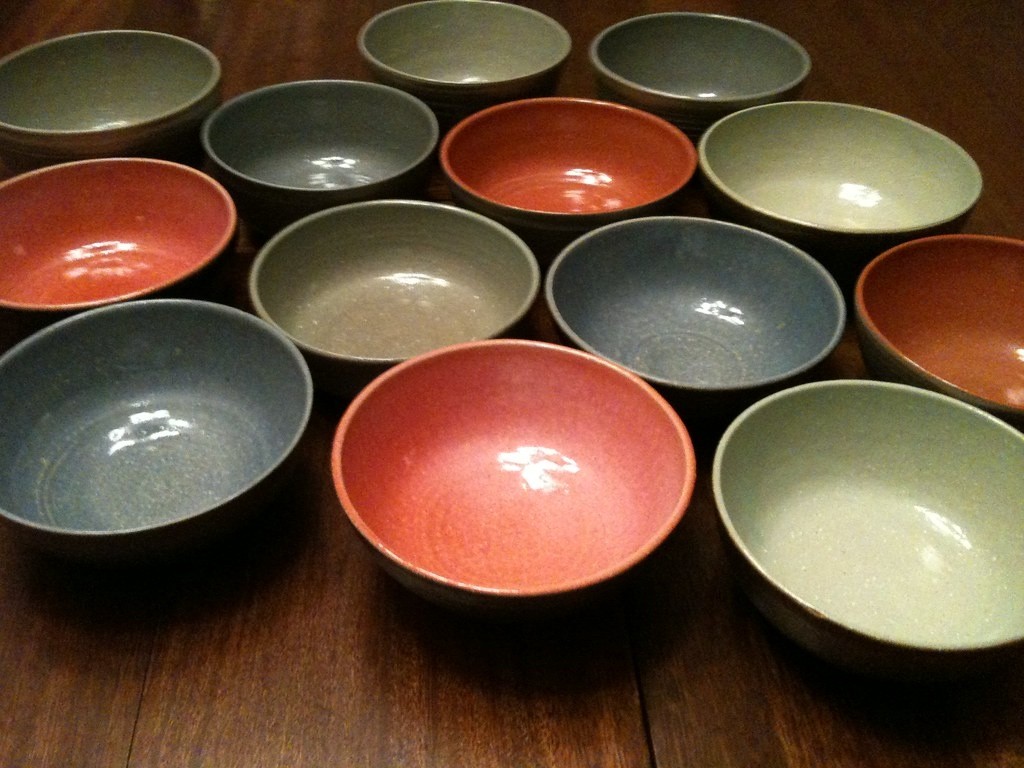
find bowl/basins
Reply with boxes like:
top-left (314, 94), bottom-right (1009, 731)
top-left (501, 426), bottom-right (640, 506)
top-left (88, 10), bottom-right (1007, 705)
top-left (544, 215), bottom-right (848, 396)
top-left (202, 79), bottom-right (438, 205)
top-left (0, 300), bottom-right (311, 540)
top-left (355, 0), bottom-right (574, 122)
top-left (246, 197), bottom-right (542, 366)
top-left (699, 101), bottom-right (986, 273)
top-left (438, 97), bottom-right (697, 240)
top-left (588, 12), bottom-right (812, 141)
top-left (712, 379), bottom-right (1024, 654)
top-left (853, 233), bottom-right (1024, 416)
top-left (330, 337), bottom-right (699, 599)
top-left (1, 29), bottom-right (223, 169)
top-left (0, 160), bottom-right (239, 312)
